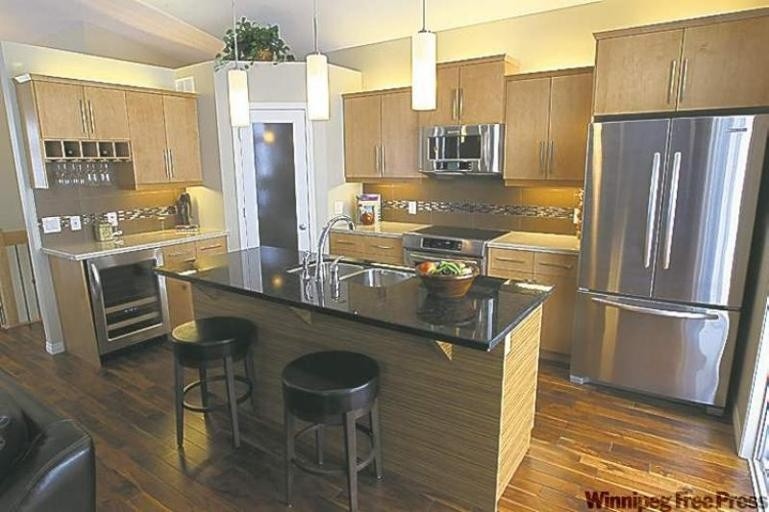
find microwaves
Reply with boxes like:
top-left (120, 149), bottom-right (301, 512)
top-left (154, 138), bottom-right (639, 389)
top-left (419, 123), bottom-right (503, 180)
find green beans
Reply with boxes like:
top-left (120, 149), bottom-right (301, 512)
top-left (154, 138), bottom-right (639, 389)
top-left (436, 260), bottom-right (461, 276)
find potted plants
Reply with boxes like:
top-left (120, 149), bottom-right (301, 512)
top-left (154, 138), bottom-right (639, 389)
top-left (212, 15), bottom-right (298, 76)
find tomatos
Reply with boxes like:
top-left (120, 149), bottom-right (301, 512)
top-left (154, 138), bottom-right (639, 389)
top-left (419, 261), bottom-right (436, 273)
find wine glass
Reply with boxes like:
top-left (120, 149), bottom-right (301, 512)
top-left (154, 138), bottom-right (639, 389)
top-left (53, 161), bottom-right (113, 187)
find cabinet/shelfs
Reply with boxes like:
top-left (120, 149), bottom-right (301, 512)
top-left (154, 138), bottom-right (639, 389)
top-left (533, 247), bottom-right (581, 368)
top-left (417, 52), bottom-right (521, 127)
top-left (324, 228), bottom-right (365, 262)
top-left (501, 64), bottom-right (596, 190)
top-left (154, 231), bottom-right (229, 342)
top-left (11, 70), bottom-right (133, 192)
top-left (340, 84), bottom-right (428, 185)
top-left (590, 5), bottom-right (769, 122)
top-left (365, 230), bottom-right (403, 266)
top-left (123, 84), bottom-right (205, 192)
top-left (486, 244), bottom-right (533, 286)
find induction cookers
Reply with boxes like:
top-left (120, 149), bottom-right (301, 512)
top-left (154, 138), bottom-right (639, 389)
top-left (400, 224), bottom-right (507, 257)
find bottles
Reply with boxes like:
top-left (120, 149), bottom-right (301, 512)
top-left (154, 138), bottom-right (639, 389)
top-left (111, 219), bottom-right (118, 236)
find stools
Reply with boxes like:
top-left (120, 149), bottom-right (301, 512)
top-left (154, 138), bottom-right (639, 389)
top-left (281, 348), bottom-right (384, 512)
top-left (170, 316), bottom-right (263, 452)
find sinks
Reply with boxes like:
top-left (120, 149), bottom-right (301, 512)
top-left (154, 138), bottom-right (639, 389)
top-left (285, 259), bottom-right (369, 278)
top-left (341, 266), bottom-right (416, 289)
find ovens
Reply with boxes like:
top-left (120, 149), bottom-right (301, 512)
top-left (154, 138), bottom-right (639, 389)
top-left (404, 250), bottom-right (485, 275)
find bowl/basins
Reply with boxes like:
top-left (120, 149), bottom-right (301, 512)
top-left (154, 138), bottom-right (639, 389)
top-left (411, 262), bottom-right (481, 302)
top-left (417, 297), bottom-right (477, 327)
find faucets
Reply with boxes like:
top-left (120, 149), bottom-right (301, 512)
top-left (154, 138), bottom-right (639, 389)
top-left (314, 279), bottom-right (326, 308)
top-left (315, 214), bottom-right (355, 279)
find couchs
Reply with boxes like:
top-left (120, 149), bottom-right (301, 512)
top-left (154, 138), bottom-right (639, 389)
top-left (0, 364), bottom-right (98, 512)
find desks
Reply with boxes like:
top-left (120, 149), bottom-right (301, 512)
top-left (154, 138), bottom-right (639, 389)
top-left (150, 244), bottom-right (561, 512)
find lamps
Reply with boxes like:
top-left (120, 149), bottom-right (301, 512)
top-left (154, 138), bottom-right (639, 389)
top-left (408, 1), bottom-right (441, 114)
top-left (305, 0), bottom-right (332, 122)
top-left (224, 1), bottom-right (253, 129)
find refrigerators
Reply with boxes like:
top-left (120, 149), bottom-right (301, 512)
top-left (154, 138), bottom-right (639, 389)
top-left (567, 112), bottom-right (769, 418)
